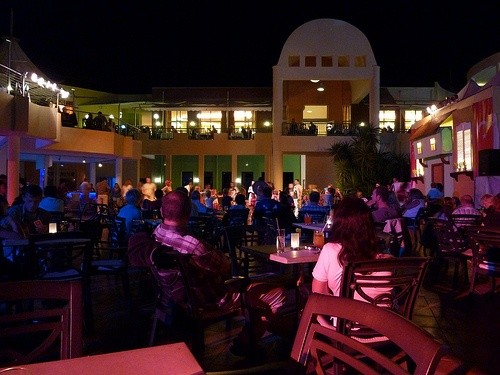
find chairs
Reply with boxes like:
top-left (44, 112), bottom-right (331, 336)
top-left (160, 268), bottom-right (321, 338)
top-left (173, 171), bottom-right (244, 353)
top-left (0, 208), bottom-right (500, 375)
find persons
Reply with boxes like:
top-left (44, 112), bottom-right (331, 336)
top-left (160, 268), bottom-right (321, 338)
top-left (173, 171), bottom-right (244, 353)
top-left (0, 185), bottom-right (50, 278)
top-left (39, 178), bottom-right (297, 241)
top-left (290, 119), bottom-right (316, 136)
top-left (300, 183), bottom-right (500, 264)
top-left (62, 105), bottom-right (115, 132)
top-left (241, 126), bottom-right (252, 140)
top-left (152, 191), bottom-right (287, 359)
top-left (311, 198), bottom-right (394, 342)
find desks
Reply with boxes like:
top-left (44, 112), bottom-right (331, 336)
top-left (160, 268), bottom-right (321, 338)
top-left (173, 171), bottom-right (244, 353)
top-left (292, 223), bottom-right (332, 231)
top-left (0, 342), bottom-right (207, 375)
top-left (241, 243), bottom-right (322, 312)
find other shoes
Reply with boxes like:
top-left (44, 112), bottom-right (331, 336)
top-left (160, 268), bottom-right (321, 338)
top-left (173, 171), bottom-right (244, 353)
top-left (230, 339), bottom-right (266, 363)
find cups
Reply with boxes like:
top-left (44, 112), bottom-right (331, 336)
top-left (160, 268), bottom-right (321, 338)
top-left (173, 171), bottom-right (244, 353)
top-left (329, 209), bottom-right (335, 222)
top-left (275, 229), bottom-right (285, 254)
top-left (304, 214), bottom-right (311, 226)
top-left (313, 230), bottom-right (324, 250)
